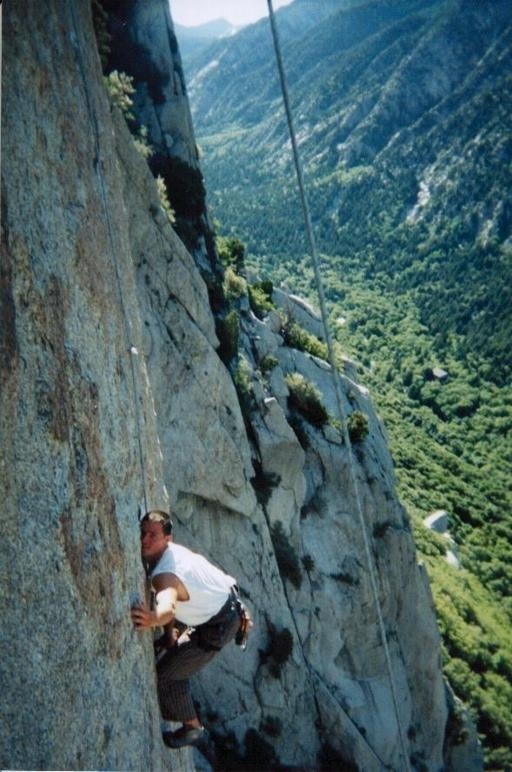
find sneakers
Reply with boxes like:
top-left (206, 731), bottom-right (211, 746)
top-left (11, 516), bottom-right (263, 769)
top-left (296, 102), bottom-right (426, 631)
top-left (164, 724), bottom-right (209, 748)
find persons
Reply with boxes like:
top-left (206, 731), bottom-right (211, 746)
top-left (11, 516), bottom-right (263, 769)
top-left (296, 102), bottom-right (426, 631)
top-left (132, 511), bottom-right (253, 749)
